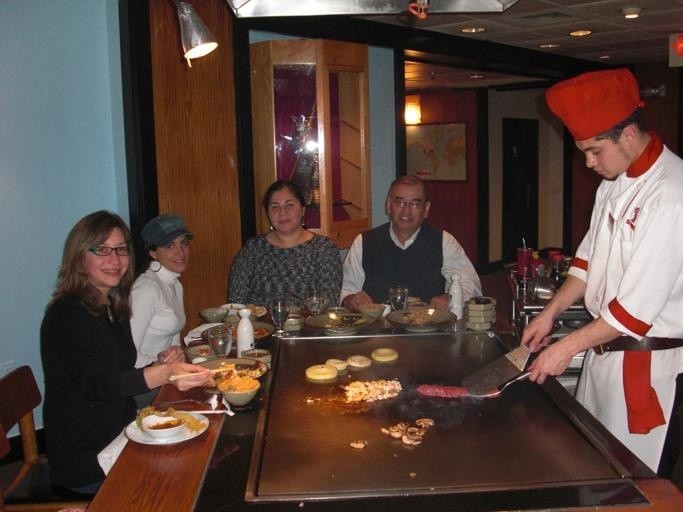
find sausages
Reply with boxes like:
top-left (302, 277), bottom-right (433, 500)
top-left (417, 384), bottom-right (500, 397)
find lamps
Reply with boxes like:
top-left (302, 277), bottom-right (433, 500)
top-left (167, 2), bottom-right (218, 67)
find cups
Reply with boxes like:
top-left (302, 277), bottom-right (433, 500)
top-left (387, 288), bottom-right (408, 310)
top-left (464, 295), bottom-right (497, 331)
top-left (239, 348), bottom-right (271, 370)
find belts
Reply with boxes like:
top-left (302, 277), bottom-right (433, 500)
top-left (591, 335), bottom-right (682, 355)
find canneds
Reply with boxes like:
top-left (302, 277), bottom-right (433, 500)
top-left (518, 247), bottom-right (532, 284)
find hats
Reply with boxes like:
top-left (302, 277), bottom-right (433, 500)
top-left (544, 68), bottom-right (645, 141)
top-left (139, 214), bottom-right (194, 252)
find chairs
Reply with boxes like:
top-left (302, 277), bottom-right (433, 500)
top-left (0, 363), bottom-right (99, 512)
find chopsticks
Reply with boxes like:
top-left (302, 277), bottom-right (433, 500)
top-left (168, 367), bottom-right (230, 381)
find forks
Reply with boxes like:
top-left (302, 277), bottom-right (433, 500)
top-left (159, 396), bottom-right (210, 406)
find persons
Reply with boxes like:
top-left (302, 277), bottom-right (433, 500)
top-left (338, 174), bottom-right (483, 320)
top-left (519, 106), bottom-right (681, 478)
top-left (224, 178), bottom-right (341, 308)
top-left (127, 211), bottom-right (195, 414)
top-left (38, 208), bottom-right (211, 496)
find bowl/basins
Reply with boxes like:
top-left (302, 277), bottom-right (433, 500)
top-left (141, 413), bottom-right (187, 438)
top-left (216, 377), bottom-right (261, 407)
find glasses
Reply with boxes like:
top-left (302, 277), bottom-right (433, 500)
top-left (392, 199), bottom-right (426, 209)
top-left (86, 244), bottom-right (131, 256)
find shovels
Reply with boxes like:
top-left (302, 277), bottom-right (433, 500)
top-left (463, 321), bottom-right (563, 398)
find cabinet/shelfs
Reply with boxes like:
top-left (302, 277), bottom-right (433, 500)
top-left (251, 38), bottom-right (371, 249)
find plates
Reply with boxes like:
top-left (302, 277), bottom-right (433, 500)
top-left (183, 294), bottom-right (456, 365)
top-left (190, 357), bottom-right (268, 388)
top-left (124, 412), bottom-right (209, 446)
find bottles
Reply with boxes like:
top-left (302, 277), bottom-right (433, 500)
top-left (516, 238), bottom-right (572, 288)
top-left (448, 273), bottom-right (463, 321)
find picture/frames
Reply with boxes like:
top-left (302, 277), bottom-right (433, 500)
top-left (405, 120), bottom-right (470, 183)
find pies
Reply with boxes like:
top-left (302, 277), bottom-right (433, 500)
top-left (306, 348), bottom-right (397, 380)
